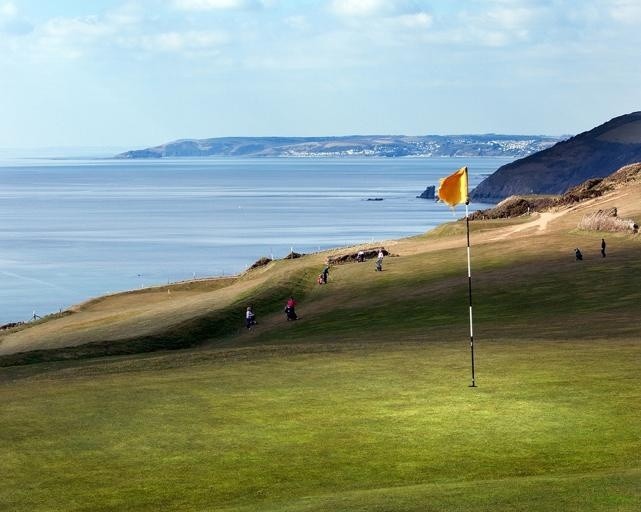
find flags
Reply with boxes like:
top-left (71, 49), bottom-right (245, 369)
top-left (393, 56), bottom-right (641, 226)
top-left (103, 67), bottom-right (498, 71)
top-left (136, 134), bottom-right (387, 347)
top-left (438, 166), bottom-right (471, 209)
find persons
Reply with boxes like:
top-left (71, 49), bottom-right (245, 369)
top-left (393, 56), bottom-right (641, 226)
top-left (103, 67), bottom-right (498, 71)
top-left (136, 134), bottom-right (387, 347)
top-left (246, 306), bottom-right (258, 328)
top-left (377, 249), bottom-right (384, 271)
top-left (284, 295), bottom-right (298, 322)
top-left (602, 238), bottom-right (607, 258)
top-left (575, 247), bottom-right (583, 260)
top-left (358, 250), bottom-right (365, 263)
top-left (323, 265), bottom-right (331, 285)
